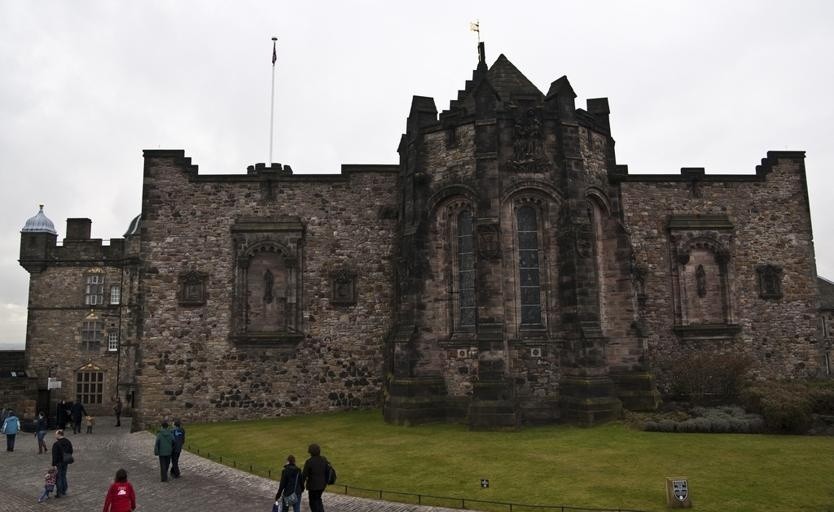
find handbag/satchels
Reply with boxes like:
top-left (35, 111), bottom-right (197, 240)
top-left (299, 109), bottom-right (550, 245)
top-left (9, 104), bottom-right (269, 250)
top-left (272, 502), bottom-right (280, 512)
top-left (62, 453), bottom-right (74, 465)
top-left (326, 464), bottom-right (336, 484)
top-left (283, 493), bottom-right (298, 507)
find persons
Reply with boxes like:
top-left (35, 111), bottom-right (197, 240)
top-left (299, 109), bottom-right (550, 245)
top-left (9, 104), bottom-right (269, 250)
top-left (52, 430), bottom-right (74, 499)
top-left (153, 421), bottom-right (177, 482)
top-left (85, 413), bottom-right (96, 435)
top-left (112, 397), bottom-right (123, 427)
top-left (55, 399), bottom-right (68, 437)
top-left (65, 397), bottom-right (74, 429)
top-left (102, 468), bottom-right (137, 512)
top-left (36, 465), bottom-right (59, 504)
top-left (170, 420), bottom-right (186, 479)
top-left (34, 411), bottom-right (48, 454)
top-left (274, 455), bottom-right (305, 512)
top-left (1, 410), bottom-right (20, 453)
top-left (301, 443), bottom-right (331, 512)
top-left (70, 398), bottom-right (89, 434)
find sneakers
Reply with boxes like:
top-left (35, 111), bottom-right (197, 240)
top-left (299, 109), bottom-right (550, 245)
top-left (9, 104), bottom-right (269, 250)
top-left (38, 491), bottom-right (66, 503)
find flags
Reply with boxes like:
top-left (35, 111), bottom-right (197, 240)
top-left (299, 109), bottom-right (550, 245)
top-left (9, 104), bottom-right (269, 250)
top-left (272, 40), bottom-right (277, 67)
top-left (470, 21), bottom-right (479, 32)
